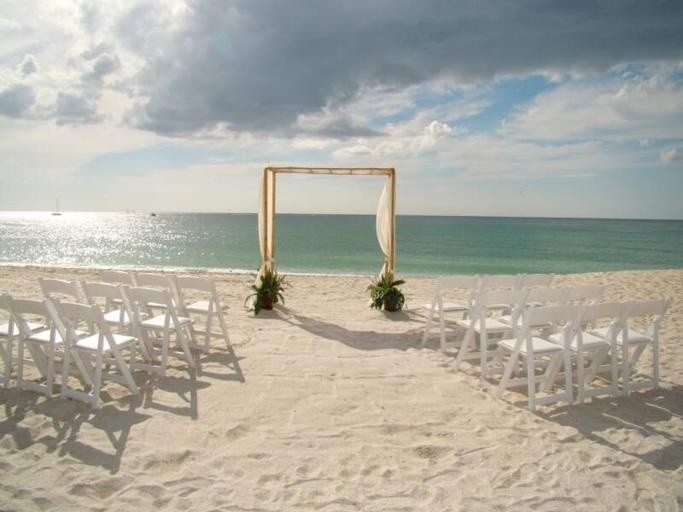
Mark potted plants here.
[364,271,406,312]
[243,264,292,314]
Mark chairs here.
[0,269,233,411]
[419,271,673,414]
